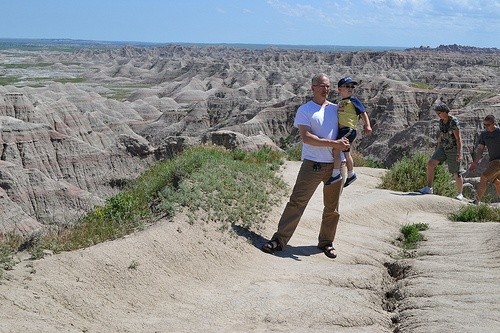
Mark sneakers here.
[326,174,342,184]
[343,173,357,187]
[419,186,433,194]
[455,192,463,201]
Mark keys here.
[313,163,320,172]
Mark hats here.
[338,76,357,88]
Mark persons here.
[467,113,500,206]
[262,73,351,258]
[418,104,464,201]
[324,77,372,188]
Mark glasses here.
[342,85,355,89]
[484,124,490,127]
[314,85,330,89]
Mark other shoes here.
[469,199,479,205]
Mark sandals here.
[262,238,283,254]
[318,243,337,258]
